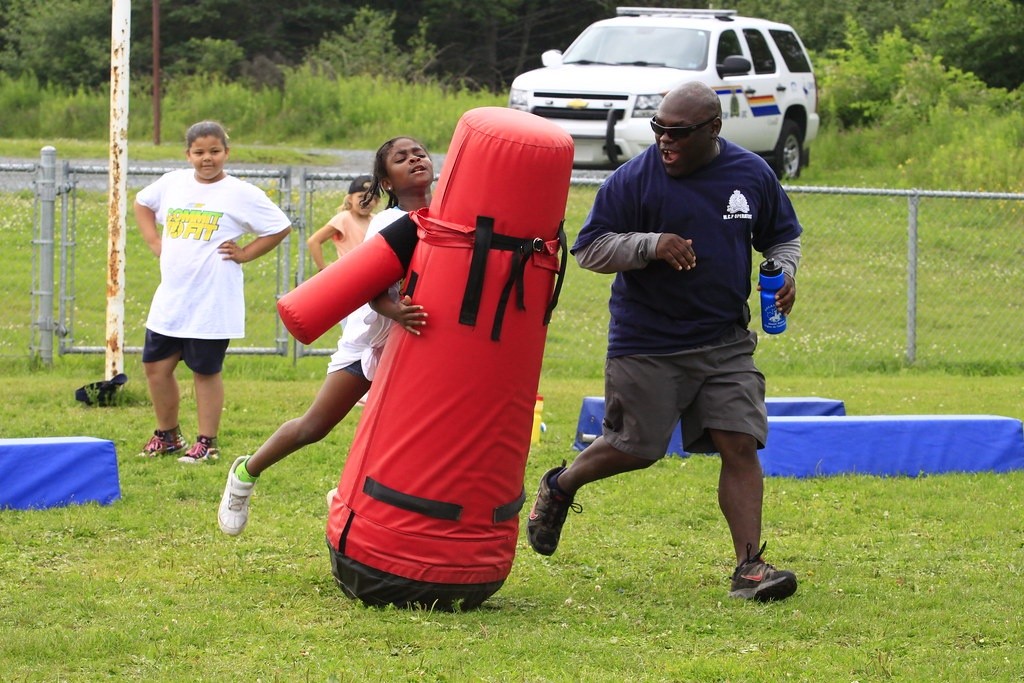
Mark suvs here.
[509,6,820,184]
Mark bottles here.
[759,258,787,334]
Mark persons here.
[218,137,434,536]
[133,121,293,462]
[528,81,804,604]
[306,175,382,406]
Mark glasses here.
[650,115,717,138]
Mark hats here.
[348,175,380,195]
[76,374,127,407]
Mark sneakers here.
[327,488,338,507]
[528,460,583,555]
[178,435,220,463]
[729,541,797,604]
[134,426,186,456]
[218,456,255,535]
